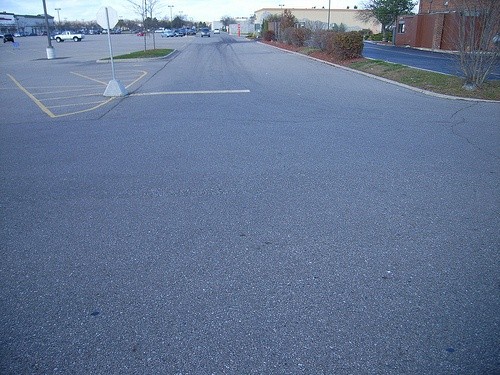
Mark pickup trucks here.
[54,31,85,43]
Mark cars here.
[136,32,144,36]
[155,28,196,38]
[80,30,121,34]
[201,29,210,38]
[3,34,14,43]
[214,29,220,34]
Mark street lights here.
[179,11,183,19]
[279,5,285,15]
[168,6,174,30]
[55,8,61,33]
